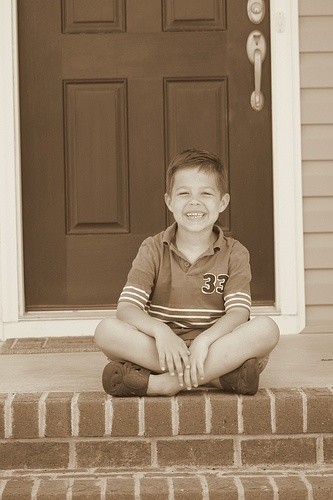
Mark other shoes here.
[216,357,259,395]
[101,359,151,398]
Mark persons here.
[92,146,280,399]
